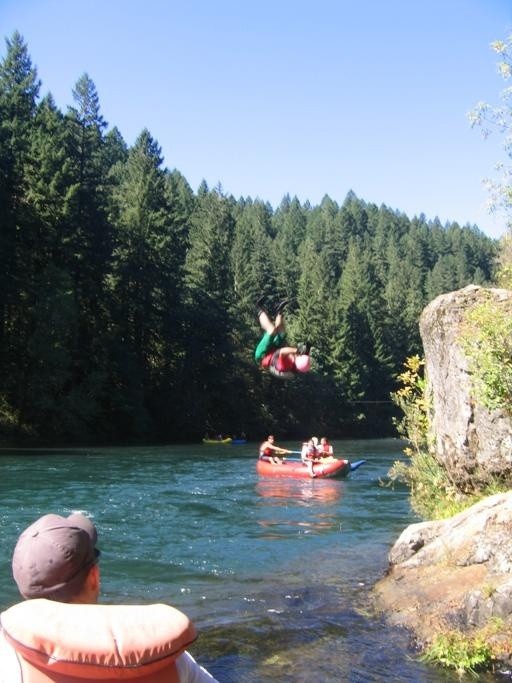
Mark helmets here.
[294,354,312,373]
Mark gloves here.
[296,340,311,354]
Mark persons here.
[319,436,333,458]
[258,435,288,465]
[301,439,321,477]
[0,513,218,682]
[252,296,313,379]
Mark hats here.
[10,510,103,605]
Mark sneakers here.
[252,293,290,317]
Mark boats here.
[202,438,247,446]
[255,459,351,480]
